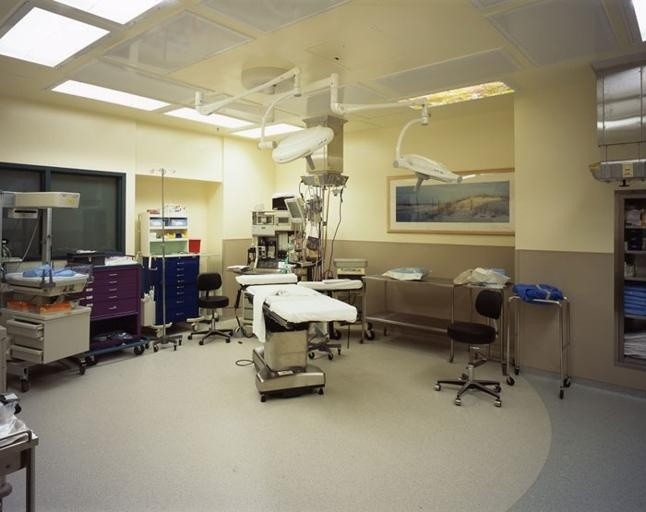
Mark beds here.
[236,271,359,401]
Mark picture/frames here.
[385,167,517,239]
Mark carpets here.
[0,314,559,512]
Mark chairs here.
[429,289,507,408]
[186,272,235,346]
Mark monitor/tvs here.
[284,198,304,224]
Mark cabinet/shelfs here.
[0,304,96,393]
[136,208,190,257]
[60,258,150,367]
[140,252,204,333]
[610,187,646,373]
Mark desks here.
[0,400,42,511]
[503,289,574,401]
[359,273,474,365]
[296,276,364,360]
[449,279,520,386]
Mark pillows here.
[235,274,301,289]
